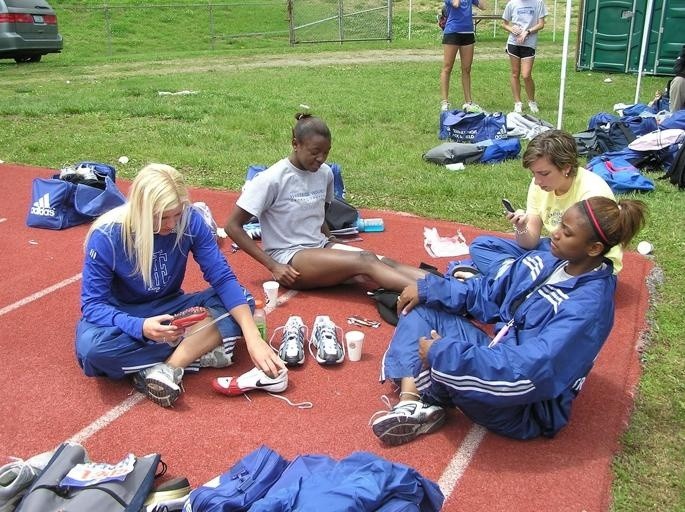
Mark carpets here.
[0,161,662,512]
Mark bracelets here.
[526,29,531,36]
[510,225,529,236]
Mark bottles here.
[356,218,385,232]
[250,300,268,340]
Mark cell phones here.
[502,199,520,220]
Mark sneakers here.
[0,452,56,512]
[164,306,209,348]
[513,102,524,113]
[200,345,236,368]
[310,315,345,366]
[441,102,451,111]
[450,264,481,282]
[277,316,306,367]
[129,362,185,409]
[211,363,289,398]
[462,103,482,112]
[372,400,448,446]
[138,477,193,512]
[528,101,539,113]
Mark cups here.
[345,330,364,363]
[636,242,654,256]
[264,281,279,308]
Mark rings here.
[396,296,401,303]
[162,337,167,341]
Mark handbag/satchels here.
[475,138,522,164]
[25,162,128,231]
[425,142,487,164]
[437,110,508,144]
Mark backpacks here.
[438,4,449,31]
[570,94,685,194]
[181,443,444,512]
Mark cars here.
[0,0,65,64]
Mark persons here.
[76,163,288,407]
[224,111,430,291]
[668,44,684,111]
[505,129,623,275]
[369,196,649,446]
[437,0,485,112]
[501,0,548,113]
